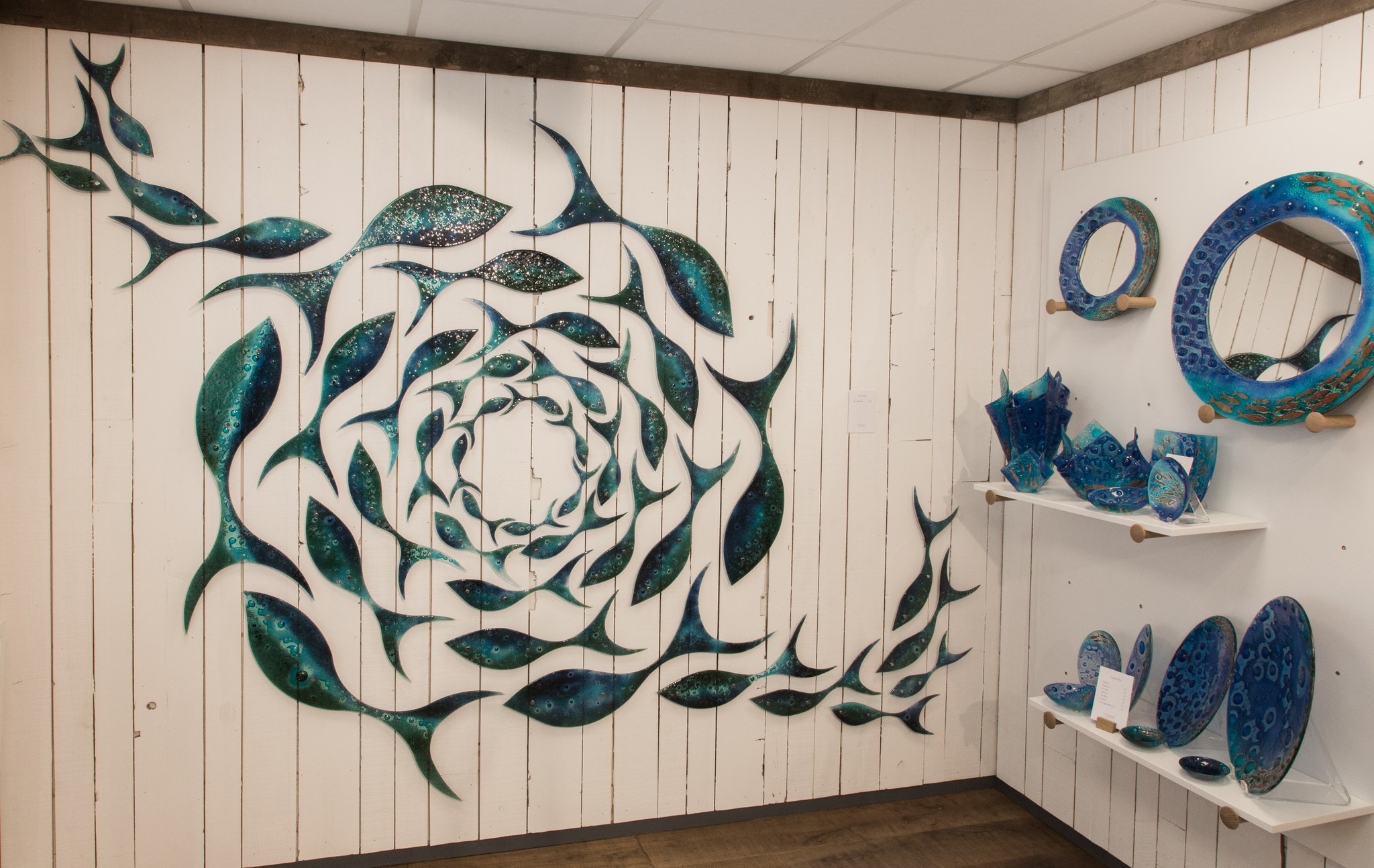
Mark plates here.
[1078,630,1121,690]
[1120,727,1164,749]
[1044,684,1096,711]
[1157,615,1237,748]
[1147,457,1189,521]
[1088,486,1147,513]
[1125,624,1153,712]
[1180,755,1231,780]
[1227,595,1315,794]
[1151,430,1218,514]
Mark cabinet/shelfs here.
[971,466,1374,868]
